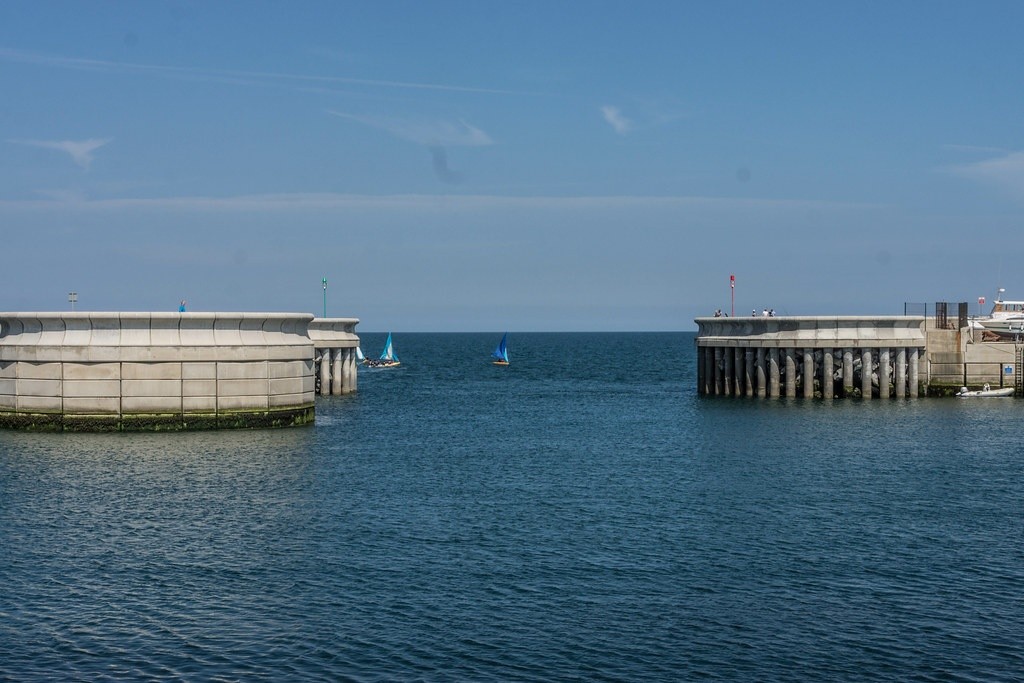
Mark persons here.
[713,308,722,317]
[751,310,756,317]
[762,309,775,317]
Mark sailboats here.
[369,332,401,367]
[491,331,510,366]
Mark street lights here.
[322,277,328,318]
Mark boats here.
[978,289,1024,333]
[955,384,1015,397]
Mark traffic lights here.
[730,276,735,317]
